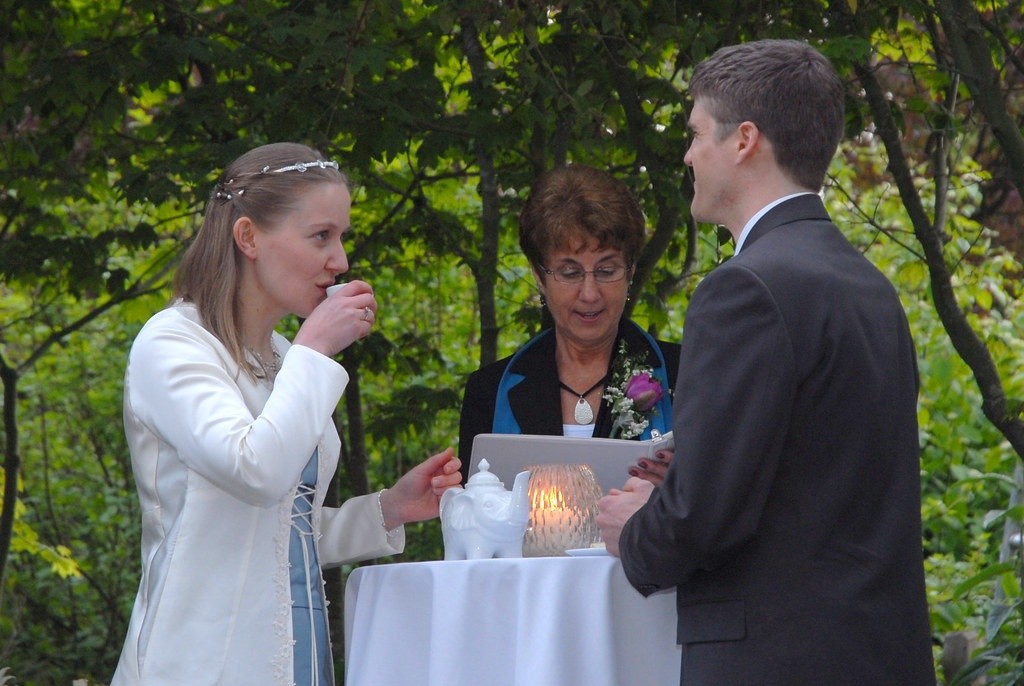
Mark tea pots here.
[438,458,531,560]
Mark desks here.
[343,557,683,686]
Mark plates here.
[565,547,614,557]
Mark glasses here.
[535,261,634,285]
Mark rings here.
[364,306,371,321]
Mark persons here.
[107,142,463,686]
[457,164,682,488]
[594,39,936,686]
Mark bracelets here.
[377,489,399,539]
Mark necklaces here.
[558,374,607,425]
[245,337,281,375]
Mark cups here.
[325,282,352,297]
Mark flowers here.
[602,338,675,441]
